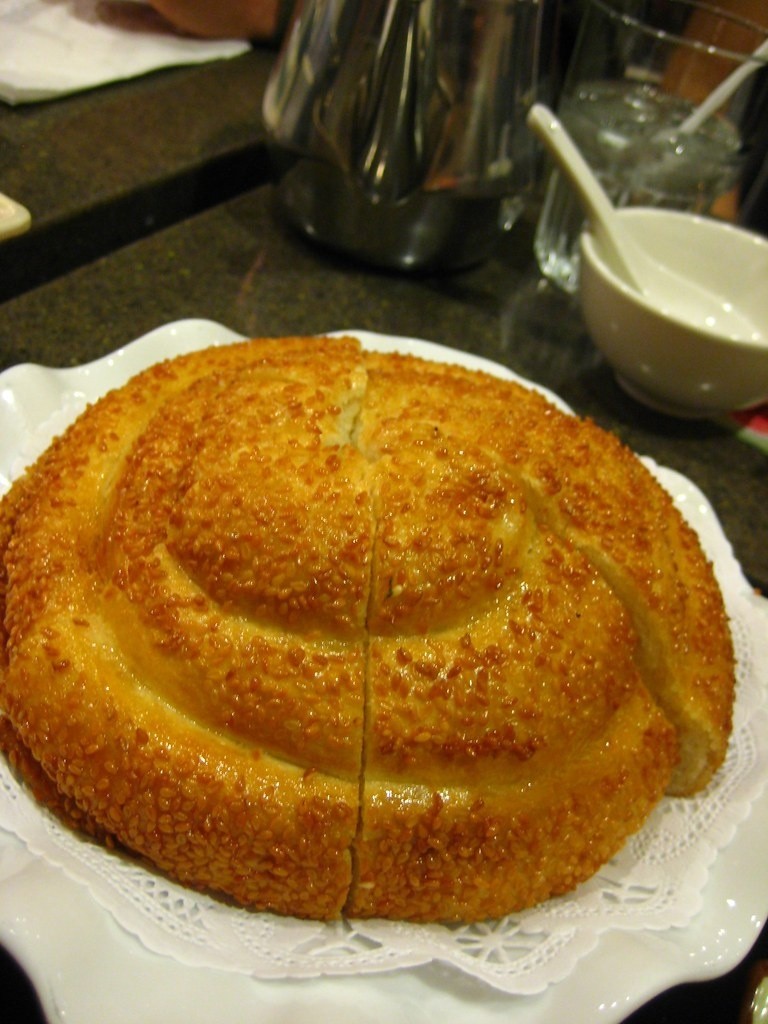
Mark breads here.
[0,331,736,926]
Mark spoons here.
[528,104,760,345]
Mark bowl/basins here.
[580,205,767,421]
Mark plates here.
[0,316,767,1024]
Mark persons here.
[44,0,280,41]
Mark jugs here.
[263,0,544,276]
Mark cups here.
[532,0,768,303]
